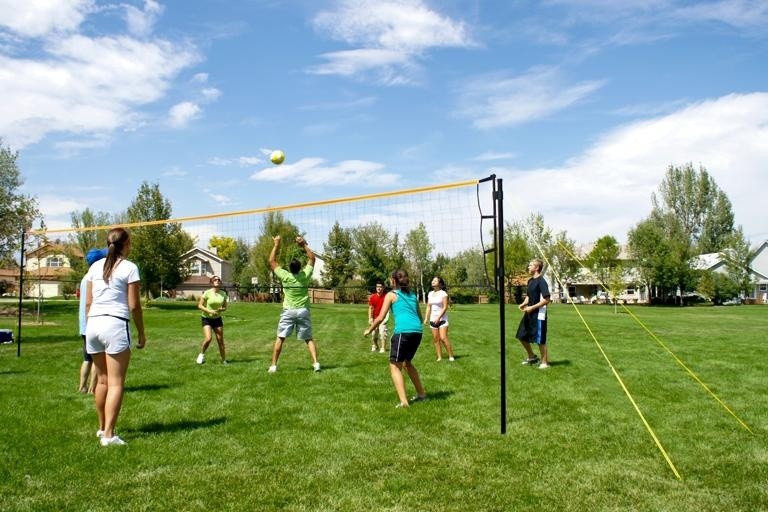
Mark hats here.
[85,247,109,267]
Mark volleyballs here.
[270,150,284,164]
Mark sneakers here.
[268,365,277,373]
[196,353,205,364]
[371,345,378,351]
[100,435,127,446]
[96,429,104,439]
[379,348,386,353]
[521,355,540,365]
[312,362,321,372]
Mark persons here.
[86,229,146,447]
[363,268,427,408]
[368,281,390,353]
[422,276,455,362]
[78,248,109,393]
[196,276,229,365]
[515,258,551,369]
[267,235,321,373]
